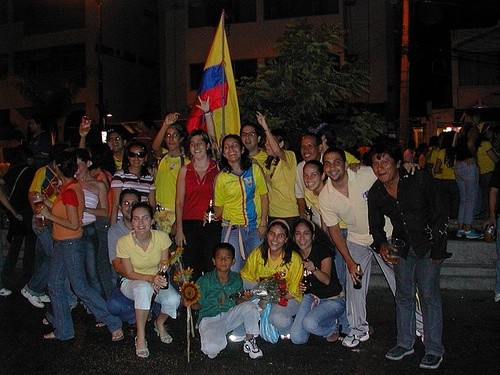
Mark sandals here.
[111,329,124,341]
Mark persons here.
[366,139,453,369]
[483,160,500,304]
[405,110,500,239]
[0,96,361,359]
[318,147,424,346]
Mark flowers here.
[229,272,290,306]
[161,247,202,363]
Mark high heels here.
[134,336,149,357]
[154,322,173,344]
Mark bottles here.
[304,206,313,221]
[302,268,310,295]
[205,199,215,227]
[159,264,170,291]
[353,264,362,289]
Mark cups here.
[81,115,94,134]
[383,237,405,264]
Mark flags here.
[185,15,241,145]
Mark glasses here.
[128,151,145,158]
[242,131,256,137]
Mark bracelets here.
[205,111,209,114]
[265,129,270,133]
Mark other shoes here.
[456,230,465,237]
[385,346,414,360]
[229,334,258,342]
[420,354,443,369]
[327,318,339,342]
[280,334,290,340]
[465,229,480,238]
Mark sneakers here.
[39,295,51,302]
[244,338,263,359]
[0,288,12,296]
[342,331,369,347]
[21,288,44,308]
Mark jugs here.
[33,193,48,229]
